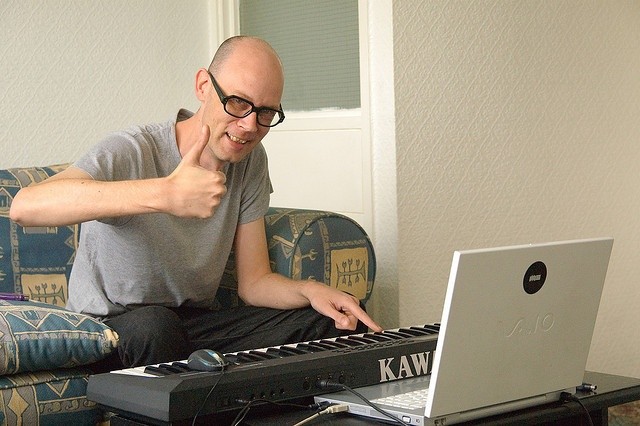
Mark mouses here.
[187,347,231,371]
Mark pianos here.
[86,323,443,421]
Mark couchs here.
[0,162,376,424]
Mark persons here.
[8,36,384,368]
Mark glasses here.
[208,69,285,127]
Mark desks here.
[97,370,639,424]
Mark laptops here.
[311,236,617,426]
